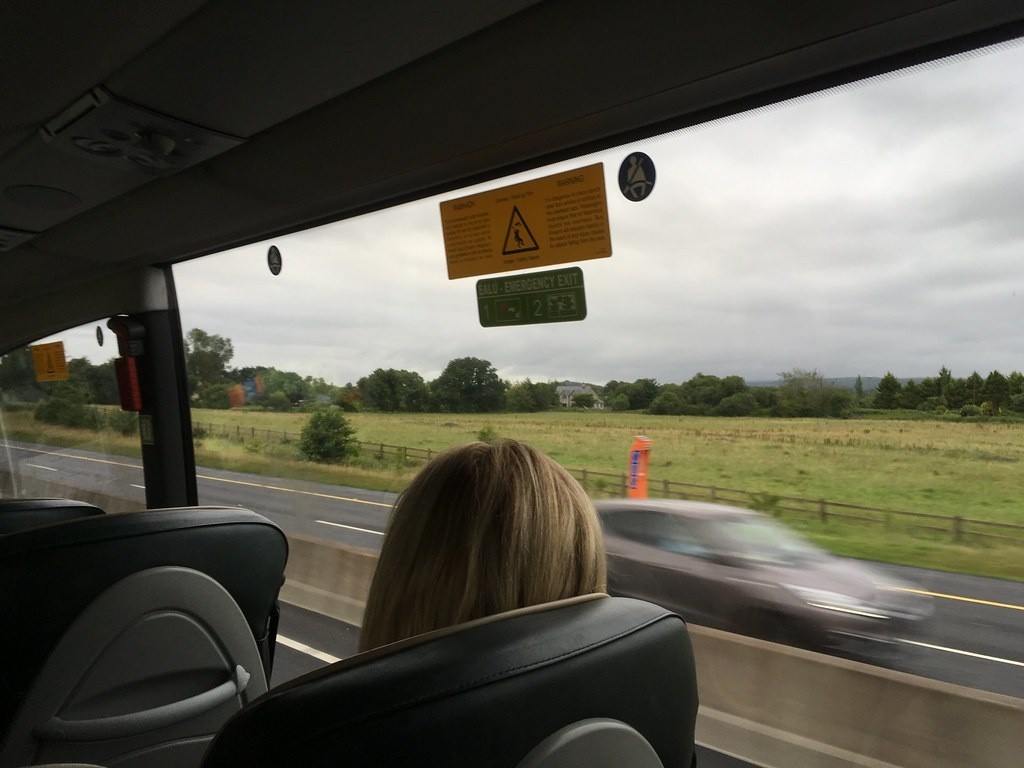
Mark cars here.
[588,496,937,662]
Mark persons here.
[358,438,608,652]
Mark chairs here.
[0,497,107,534]
[195,592,699,768]
[0,507,290,768]
